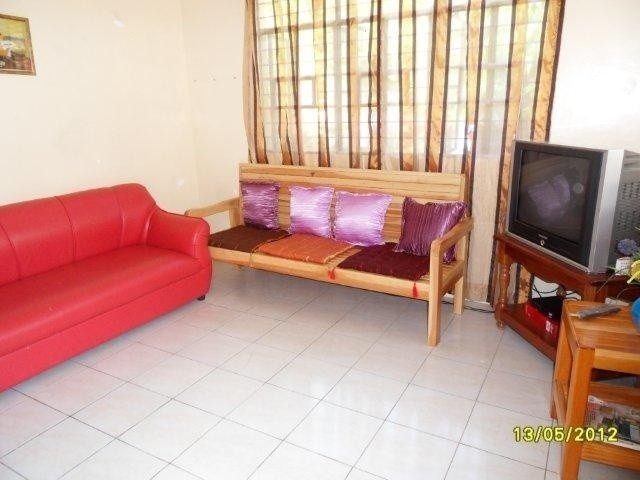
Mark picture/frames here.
[0,14,36,75]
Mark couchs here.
[185,163,474,346]
[0,183,213,393]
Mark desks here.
[550,299,640,480]
[493,233,640,381]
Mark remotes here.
[577,305,621,320]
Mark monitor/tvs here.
[504,139,640,274]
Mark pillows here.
[333,191,393,247]
[240,180,282,233]
[392,197,468,264]
[286,185,335,239]
